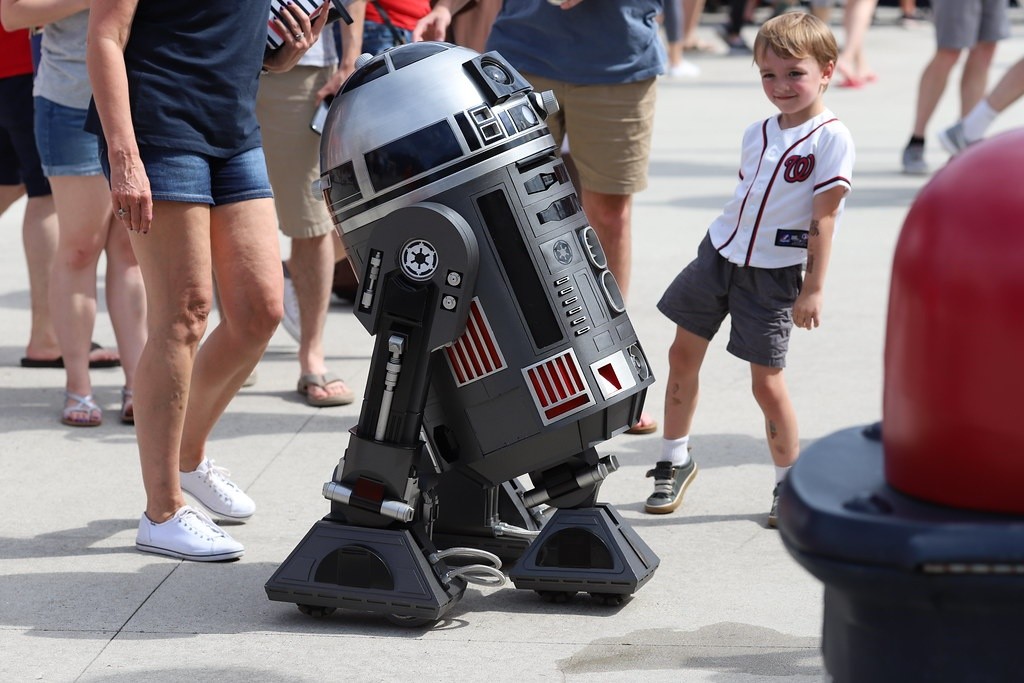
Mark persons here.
[88,0,334,562]
[937,57,1024,155]
[411,1,667,314]
[0,1,503,427]
[902,0,1009,175]
[643,12,854,528]
[657,0,916,89]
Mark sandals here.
[120,385,134,423]
[61,390,101,426]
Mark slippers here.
[20,342,120,368]
[297,372,353,406]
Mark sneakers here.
[768,482,780,527]
[135,505,244,561]
[179,456,256,520]
[645,447,699,514]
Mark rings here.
[117,209,130,217]
[295,32,305,41]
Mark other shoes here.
[715,25,752,56]
[280,260,303,344]
[670,60,697,75]
[903,15,931,31]
[938,119,985,158]
[835,73,875,87]
[902,144,927,174]
